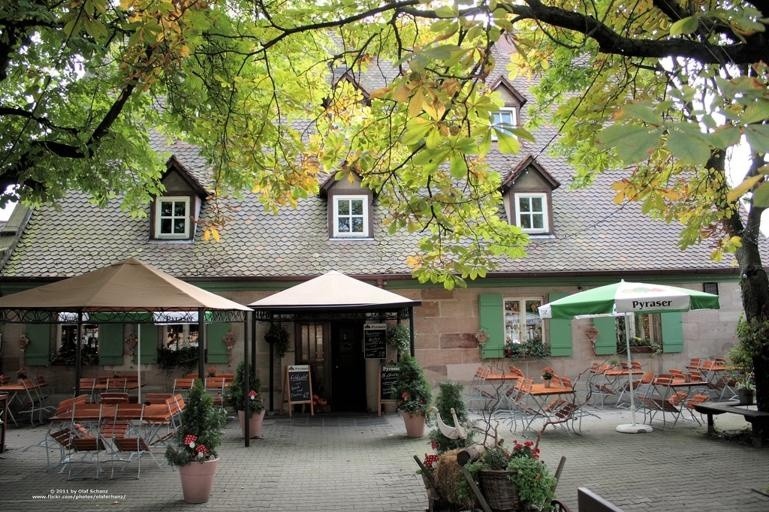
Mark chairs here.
[0,369,236,481]
[472,356,754,437]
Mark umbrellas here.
[538,278,721,427]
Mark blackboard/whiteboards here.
[289,372,310,401]
[364,330,387,359]
[379,364,401,403]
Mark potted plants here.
[232,362,265,441]
[394,349,556,511]
[165,374,228,504]
